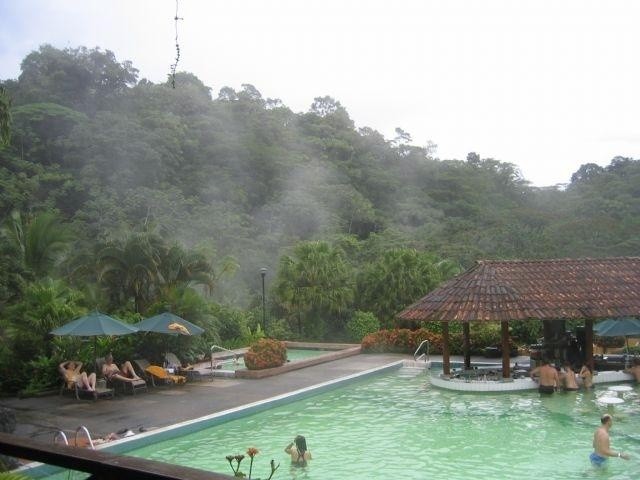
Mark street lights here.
[260,266,269,334]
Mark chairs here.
[60,359,213,403]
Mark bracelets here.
[617,452,620,457]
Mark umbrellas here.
[46,309,140,357]
[594,317,640,354]
[592,319,614,331]
[132,310,205,350]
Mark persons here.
[622,356,640,384]
[578,360,596,391]
[231,353,241,369]
[589,413,632,471]
[101,353,141,383]
[528,361,560,397]
[58,360,97,392]
[558,360,579,392]
[161,350,181,371]
[284,434,313,478]
